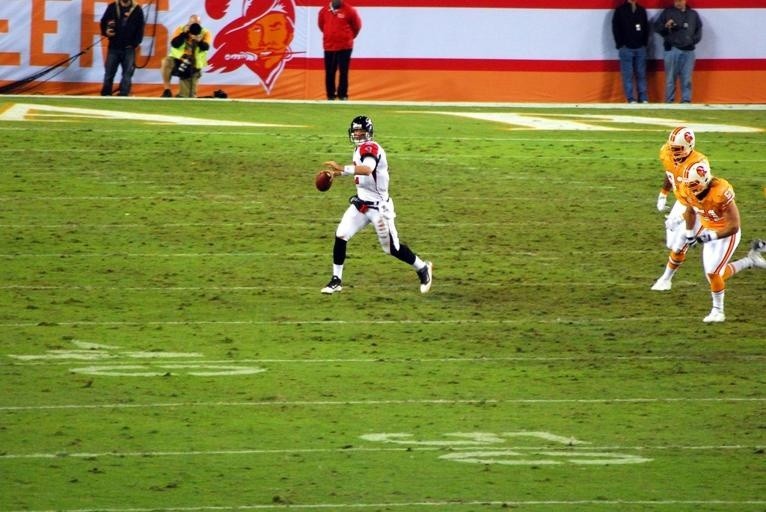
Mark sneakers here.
[650,277,672,291]
[416,260,432,293]
[163,89,172,97]
[748,248,766,267]
[320,276,342,293]
[702,308,725,322]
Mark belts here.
[362,200,378,205]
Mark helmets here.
[348,116,374,142]
[668,127,695,159]
[682,161,713,196]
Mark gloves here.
[657,197,670,211]
[695,234,712,244]
[664,215,682,231]
[686,236,698,246]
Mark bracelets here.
[343,165,355,175]
[340,171,348,176]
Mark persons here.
[653,1,703,104]
[318,0,362,101]
[320,116,434,296]
[649,125,711,291]
[99,1,145,97]
[213,0,295,93]
[159,15,213,99]
[678,160,766,322]
[612,1,650,104]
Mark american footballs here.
[315,172,333,192]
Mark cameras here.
[190,23,202,35]
